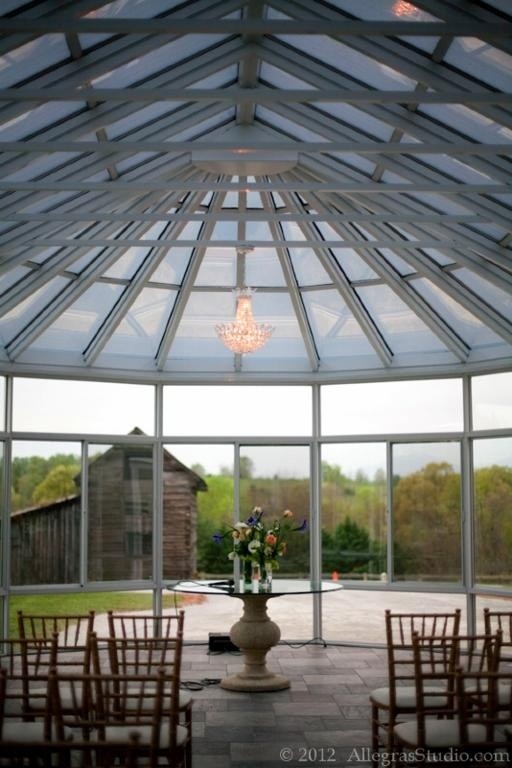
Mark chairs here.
[2,605,198,766]
[364,604,512,768]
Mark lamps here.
[213,243,276,355]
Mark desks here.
[164,576,343,696]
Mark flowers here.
[219,505,306,581]
